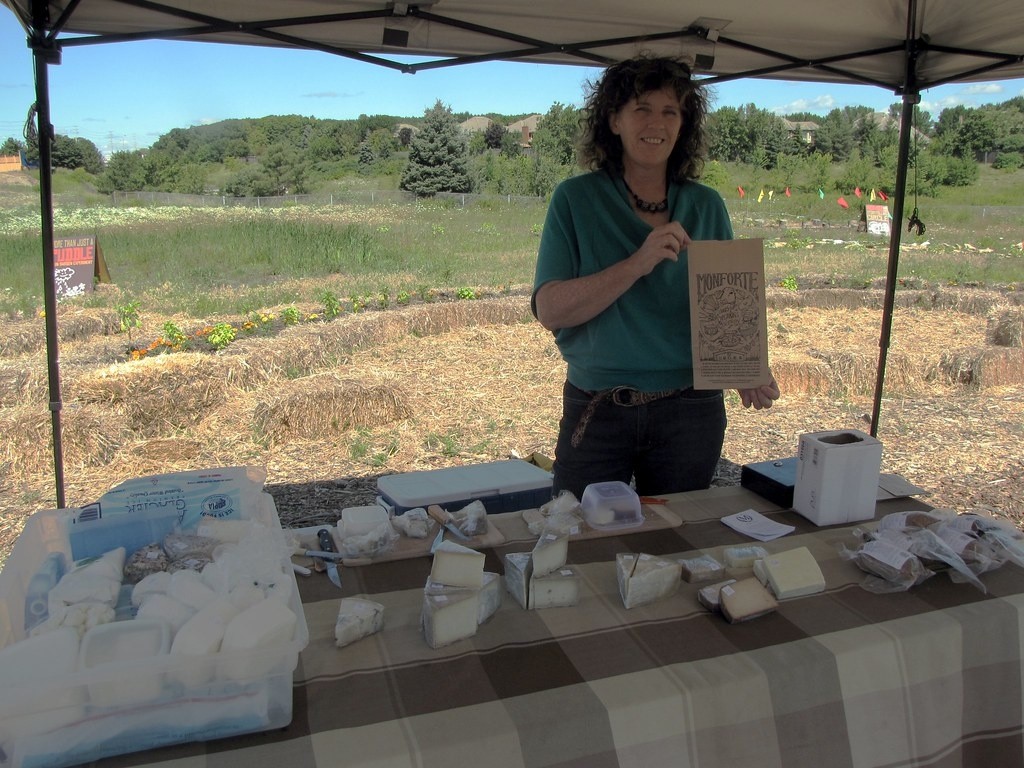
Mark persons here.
[532,58,780,498]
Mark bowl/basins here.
[582,481,645,531]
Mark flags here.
[870,189,876,202]
[758,190,764,203]
[877,191,889,201]
[738,186,744,198]
[768,191,773,200]
[855,187,862,198]
[785,187,791,197]
[818,189,824,199]
[837,197,848,208]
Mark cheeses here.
[528,494,584,537]
[419,529,584,650]
[617,546,825,623]
[336,598,385,648]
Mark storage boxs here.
[793,429,882,527]
[582,481,644,532]
[741,457,798,508]
[377,452,555,520]
[0,493,310,768]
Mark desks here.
[75,484,1024,767]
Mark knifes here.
[428,505,473,542]
[294,548,341,560]
[317,529,342,589]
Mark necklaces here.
[623,173,668,213]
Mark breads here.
[392,499,489,536]
[30,515,310,719]
